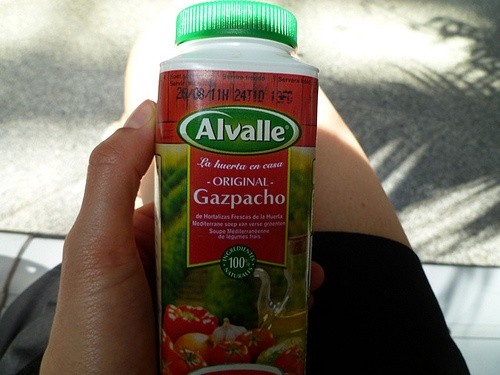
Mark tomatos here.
[161,303,304,375]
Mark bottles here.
[153,0,320,375]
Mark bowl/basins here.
[186,362,285,375]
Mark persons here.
[0,29,473,375]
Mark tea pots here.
[254,234,306,358]
